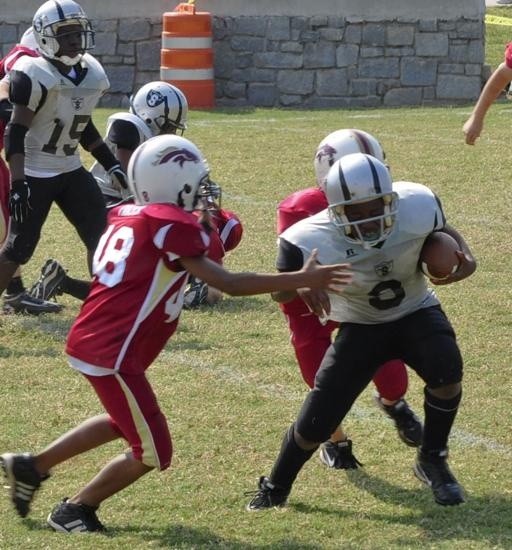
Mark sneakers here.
[3,289,62,316]
[183,276,208,310]
[413,445,465,505]
[46,497,107,532]
[244,476,290,511]
[1,452,50,518]
[319,436,362,469]
[372,384,423,447]
[30,259,68,303]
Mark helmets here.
[129,81,188,136]
[20,1,96,56]
[313,128,399,247]
[127,133,221,212]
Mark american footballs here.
[419,231,461,279]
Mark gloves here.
[106,164,129,202]
[8,180,33,224]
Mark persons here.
[90,81,188,208]
[0,0,126,299]
[30,206,243,311]
[247,152,476,515]
[0,26,61,311]
[0,135,353,533]
[463,44,512,146]
[278,129,428,469]
